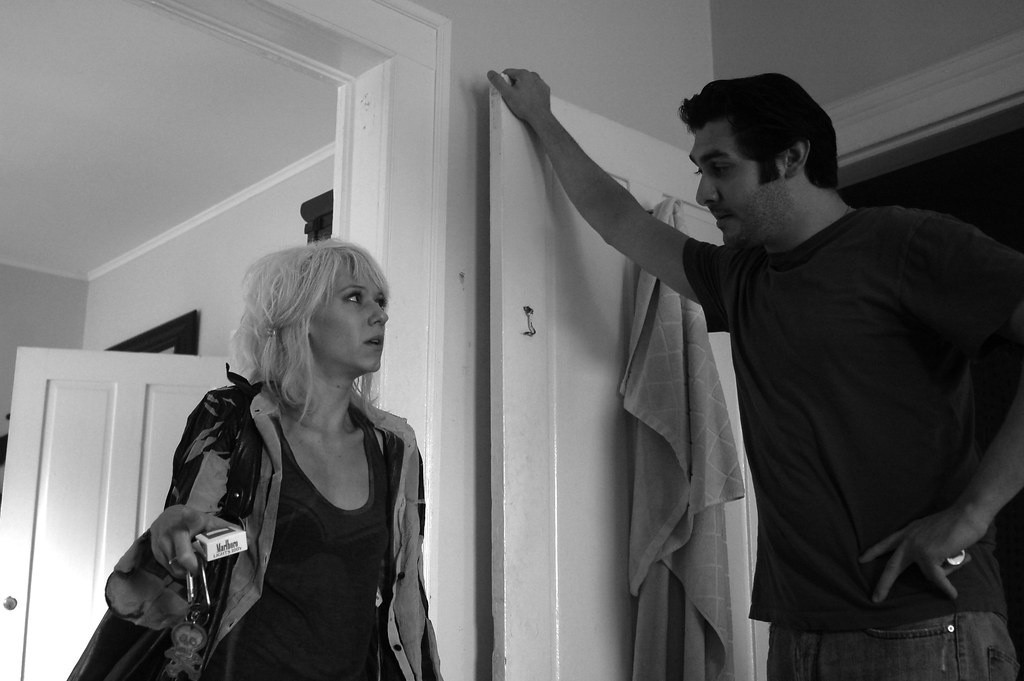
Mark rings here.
[167,556,177,565]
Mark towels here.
[618,198,746,680]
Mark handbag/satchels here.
[64,601,217,681]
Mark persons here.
[489,68,1023,681]
[107,242,443,681]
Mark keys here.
[165,552,214,680]
